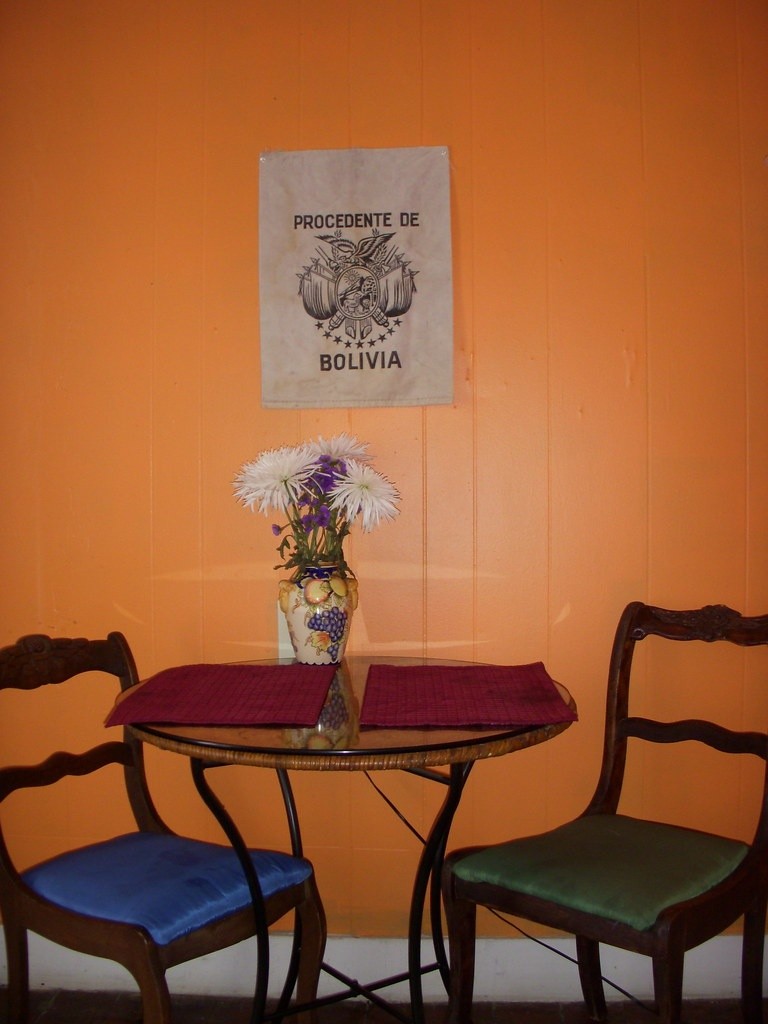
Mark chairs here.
[444,602,767,1023]
[1,632,327,1024]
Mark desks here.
[128,656,576,1024]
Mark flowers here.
[230,432,403,584]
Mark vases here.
[278,561,358,664]
[283,660,361,750]
